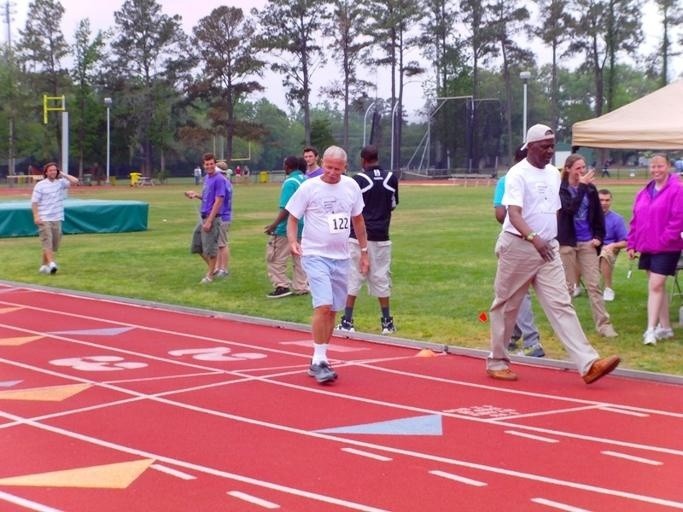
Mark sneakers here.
[380,317,397,334]
[217,270,228,278]
[573,285,582,298]
[213,268,219,275]
[200,277,214,285]
[264,285,292,299]
[642,330,656,346]
[525,345,544,358]
[38,265,49,274]
[306,361,339,384]
[602,325,618,338]
[655,322,676,340]
[602,287,614,301]
[334,318,356,333]
[48,262,57,275]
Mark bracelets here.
[360,245,368,253]
[526,230,537,241]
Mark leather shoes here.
[486,368,517,380]
[581,354,621,385]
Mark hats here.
[517,123,556,153]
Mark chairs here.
[667,251,683,305]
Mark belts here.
[504,230,532,244]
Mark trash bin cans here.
[259,171,268,184]
[83,173,92,186]
[111,175,116,186]
[130,172,142,188]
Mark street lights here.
[102,96,114,181]
[0,1,18,56]
[519,69,531,144]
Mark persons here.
[284,145,370,384]
[483,124,621,385]
[555,154,619,339]
[31,162,80,276]
[183,152,250,285]
[493,142,546,358]
[600,160,611,178]
[335,144,398,335]
[573,189,626,303]
[669,158,683,172]
[263,145,323,299]
[627,153,683,348]
